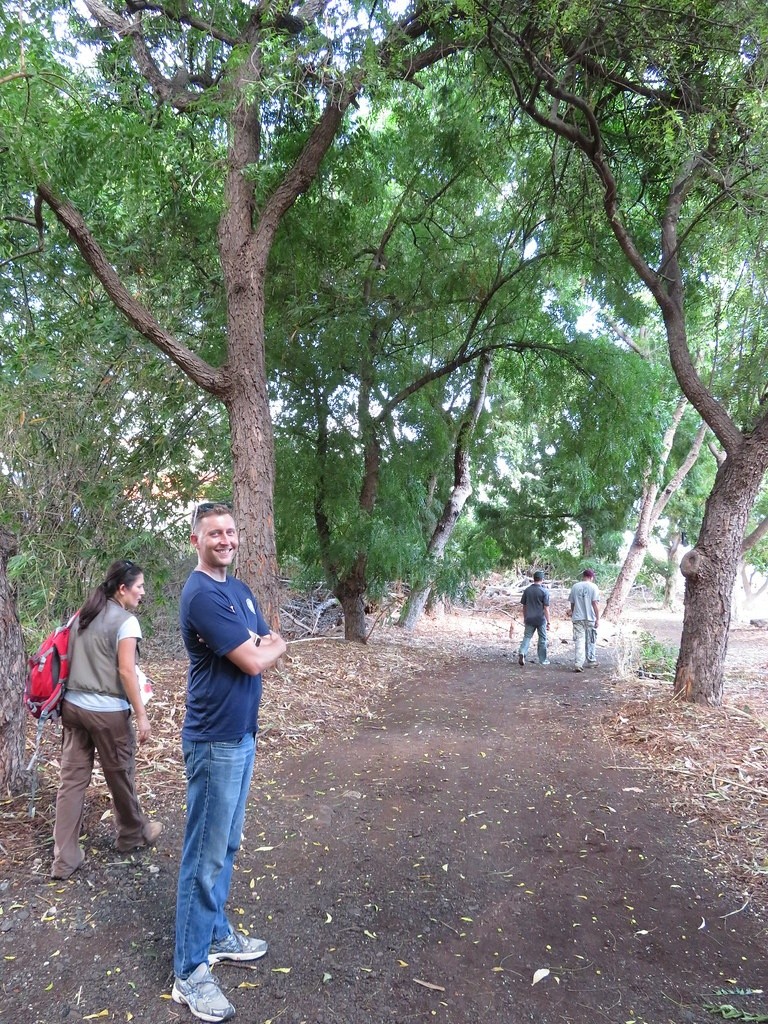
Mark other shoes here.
[142,822,163,844]
[79,849,85,863]
[576,667,585,673]
[589,663,599,669]
[540,659,551,665]
[519,654,524,666]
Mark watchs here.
[254,634,261,648]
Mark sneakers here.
[172,959,236,1022]
[207,924,270,965]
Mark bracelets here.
[547,623,550,624]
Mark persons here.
[567,569,600,672]
[518,571,550,666]
[50,560,164,880]
[170,503,287,1023]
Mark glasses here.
[193,503,234,533]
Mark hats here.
[584,570,596,578]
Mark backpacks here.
[24,607,83,722]
